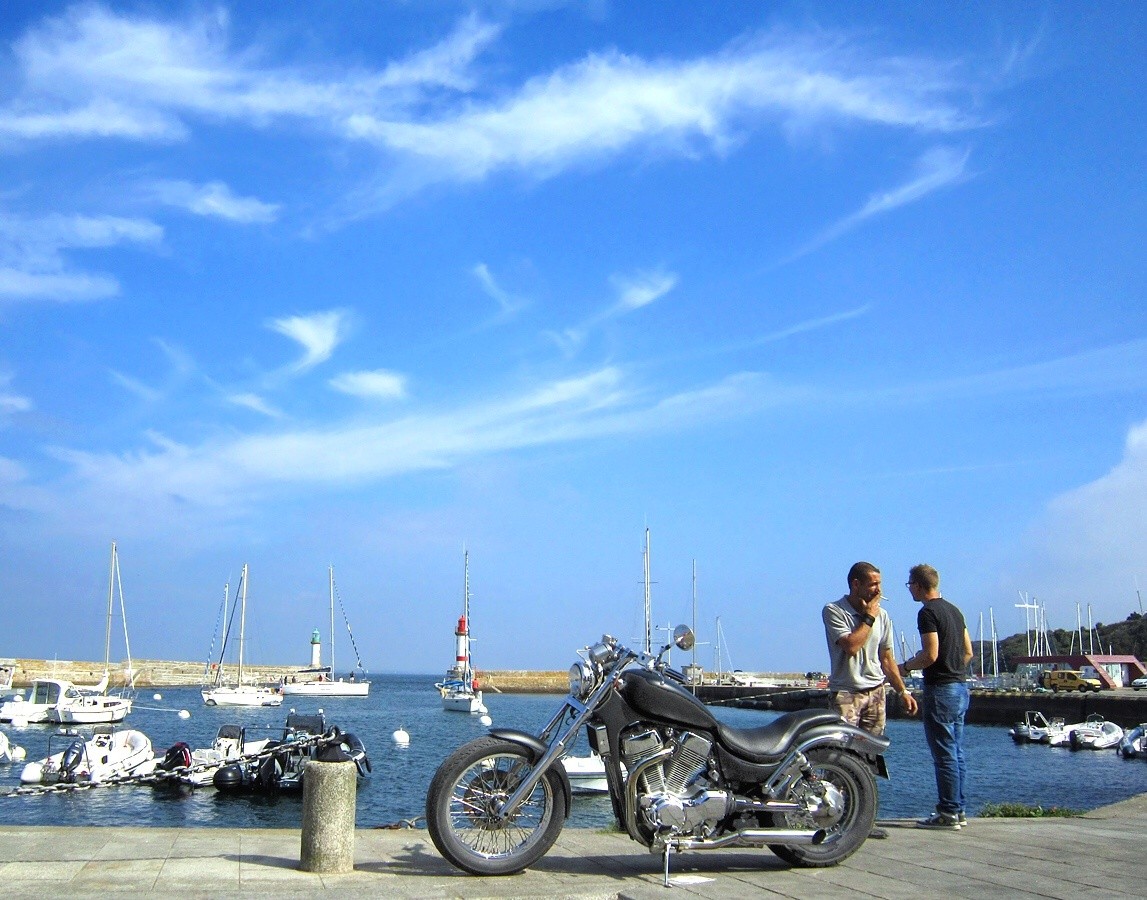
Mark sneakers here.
[916,811,962,831]
[929,811,968,825]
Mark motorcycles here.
[425,623,892,889]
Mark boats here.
[434,682,452,692]
[19,727,159,785]
[1008,718,1147,762]
[212,730,369,792]
[0,541,140,723]
[559,752,629,795]
[47,695,134,723]
[129,738,272,788]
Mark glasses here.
[905,582,915,588]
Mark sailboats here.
[277,567,369,697]
[442,549,483,713]
[201,564,284,708]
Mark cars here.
[1132,674,1147,691]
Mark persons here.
[897,564,974,830]
[822,562,918,839]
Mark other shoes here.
[869,828,889,839]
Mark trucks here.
[1043,669,1102,693]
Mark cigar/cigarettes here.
[880,596,889,601]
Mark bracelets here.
[897,689,912,703]
[903,662,911,672]
[862,614,875,627]
